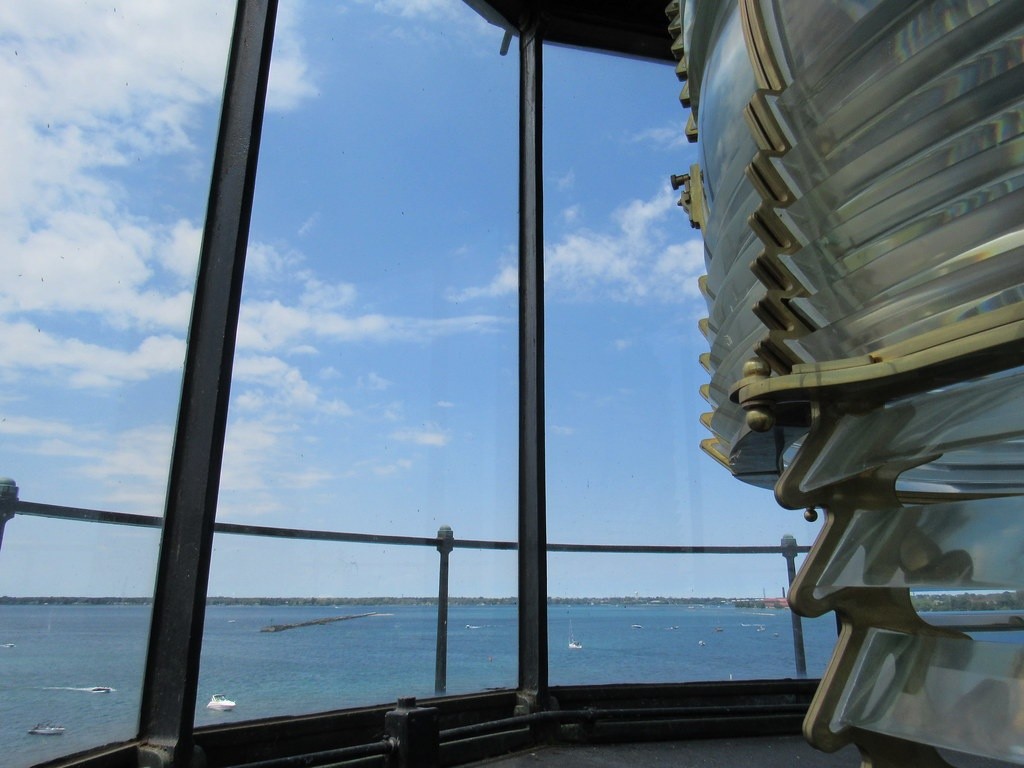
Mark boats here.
[698,640,706,645]
[568,641,583,649]
[716,626,723,632]
[466,625,470,628]
[90,688,111,694]
[206,694,236,712]
[741,612,781,636]
[27,721,66,735]
[670,624,679,629]
[2,643,14,648]
[631,624,642,628]
